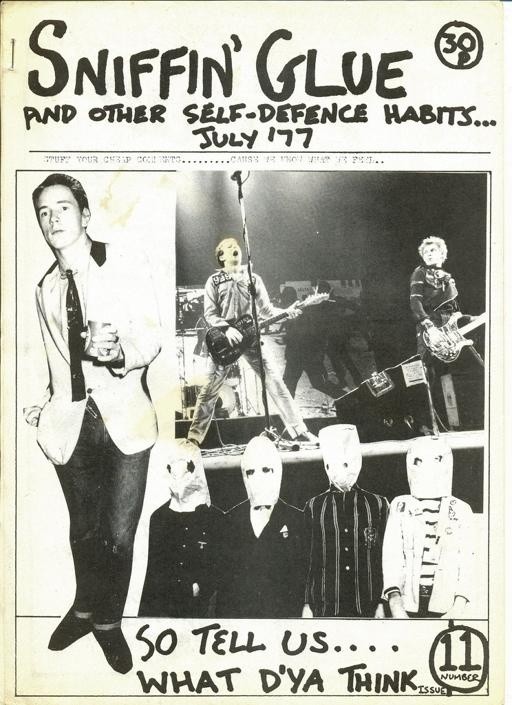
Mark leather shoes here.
[93,629,134,674]
[48,613,93,652]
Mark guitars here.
[206,291,330,367]
[418,310,486,366]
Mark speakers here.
[334,353,434,443]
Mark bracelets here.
[422,322,434,331]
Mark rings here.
[114,336,119,343]
[106,349,110,356]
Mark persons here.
[410,236,484,432]
[23,173,162,674]
[186,238,318,446]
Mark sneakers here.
[295,431,318,442]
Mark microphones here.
[230,171,242,181]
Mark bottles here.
[82,320,113,362]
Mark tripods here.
[235,181,301,451]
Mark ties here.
[65,269,86,404]
